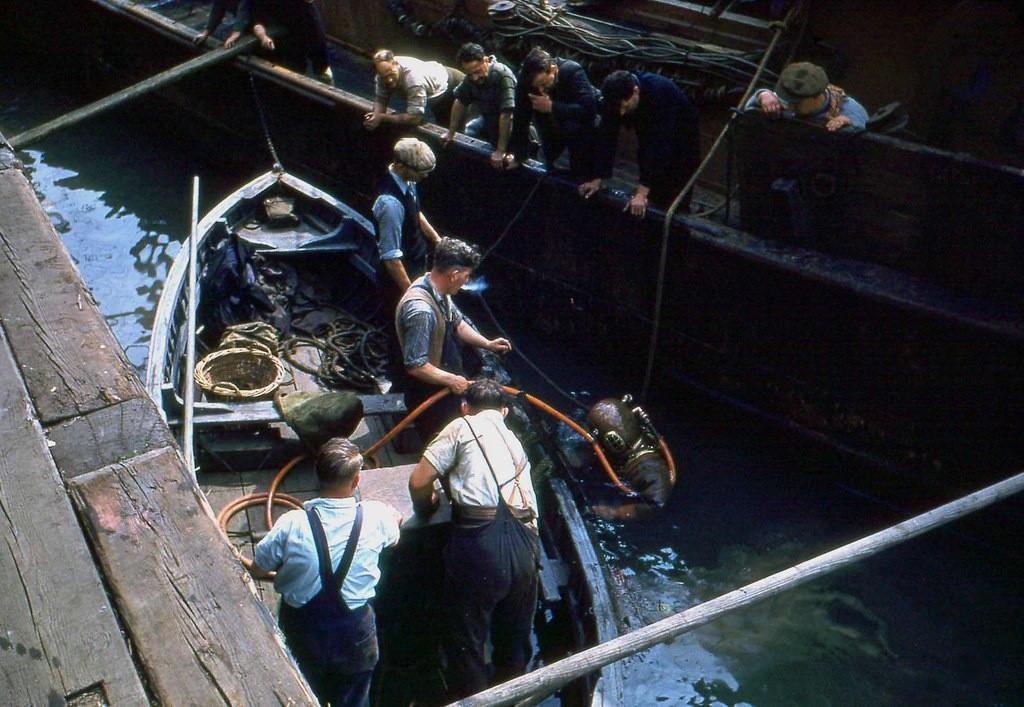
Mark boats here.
[141,159,624,706]
[89,0,1024,486]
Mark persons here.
[249,438,402,707]
[408,379,541,699]
[587,397,673,523]
[370,136,437,365]
[504,45,603,185]
[577,69,701,220]
[743,61,871,133]
[439,41,540,171]
[395,235,512,444]
[363,49,471,131]
[192,0,335,87]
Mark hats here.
[774,60,827,103]
[395,138,437,174]
